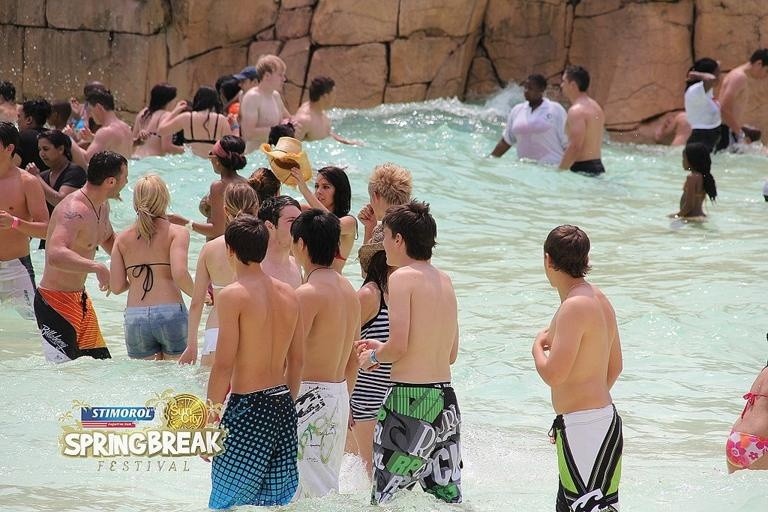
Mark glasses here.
[208,151,218,160]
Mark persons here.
[559,65,605,178]
[654,48,768,224]
[532,224,624,512]
[490,73,569,166]
[726,334,768,474]
[0,54,464,510]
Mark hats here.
[233,66,260,83]
[520,74,546,90]
[262,137,311,183]
[358,224,399,274]
